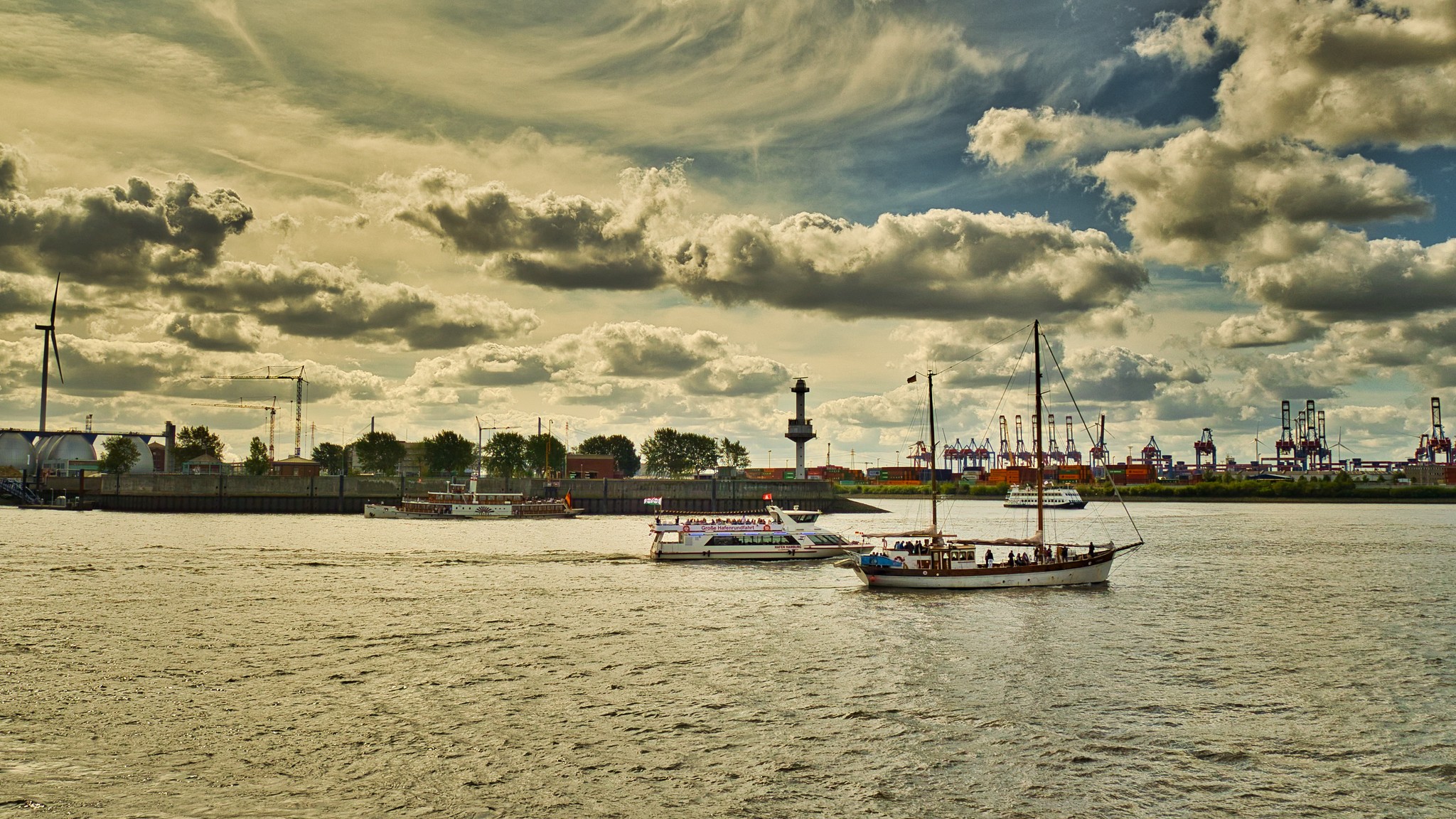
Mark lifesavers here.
[893,556,903,566]
[764,524,770,531]
[683,526,690,532]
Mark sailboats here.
[833,320,1149,588]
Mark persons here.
[985,545,1068,569]
[869,539,925,570]
[520,493,566,507]
[1088,542,1096,560]
[655,514,772,527]
[429,504,453,515]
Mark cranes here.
[200,365,310,458]
[191,396,281,476]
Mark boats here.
[1004,480,1089,509]
[364,473,584,520]
[644,493,876,560]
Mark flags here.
[644,497,655,505]
[860,532,864,535]
[650,497,662,506]
[565,488,572,510]
[855,532,859,534]
[763,494,772,500]
[907,374,917,384]
